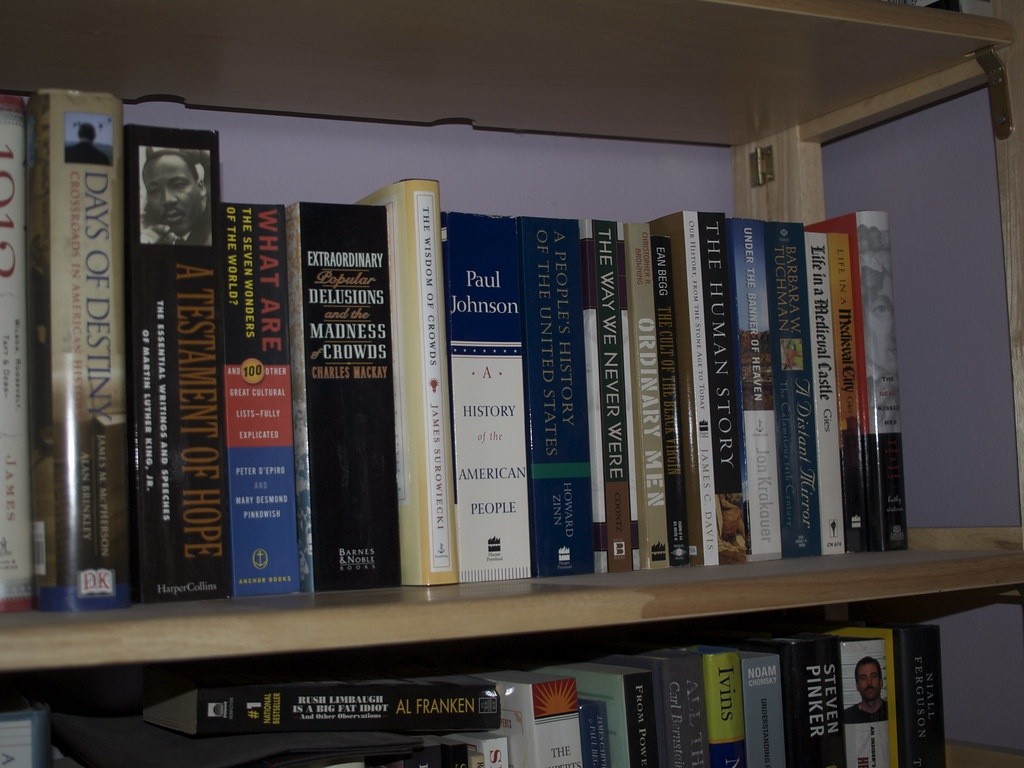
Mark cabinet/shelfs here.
[0,0,1024,768]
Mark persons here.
[142,151,213,246]
[846,657,888,723]
[65,122,112,165]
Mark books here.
[0,625,943,768]
[0,89,907,608]
